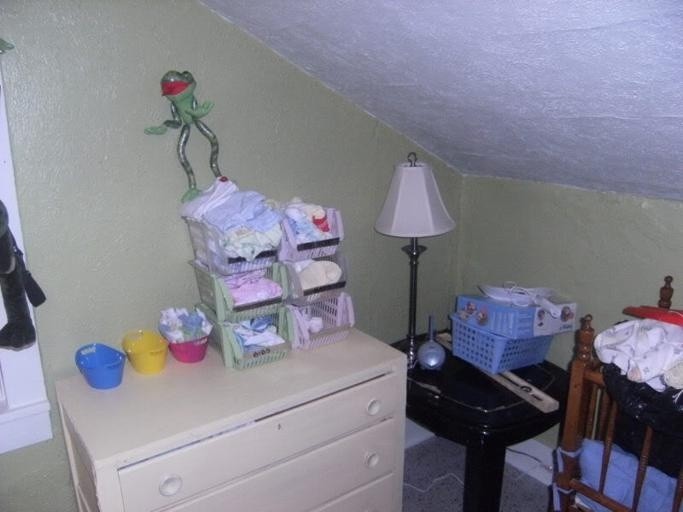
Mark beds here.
[548,273,683,512]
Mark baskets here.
[449,313,553,374]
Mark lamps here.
[373,152,457,370]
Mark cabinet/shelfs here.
[53,327,408,512]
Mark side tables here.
[389,330,572,511]
